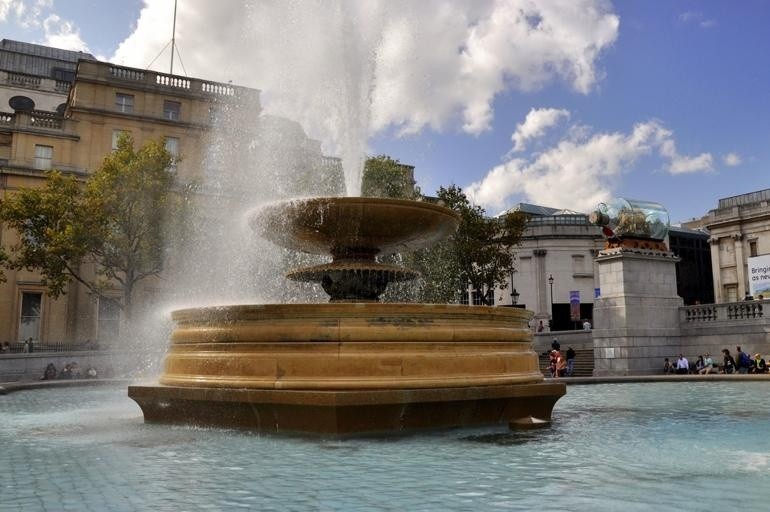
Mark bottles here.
[589,196,671,240]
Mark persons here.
[742,290,756,318]
[43,361,98,379]
[757,295,764,316]
[29,336,33,352]
[2,341,10,352]
[23,340,29,353]
[546,337,576,378]
[582,318,591,330]
[537,320,544,332]
[663,345,770,374]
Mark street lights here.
[510,288,519,306]
[548,274,553,303]
